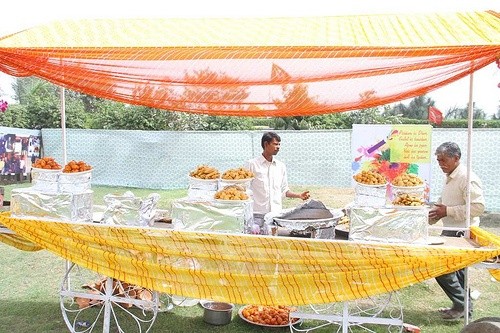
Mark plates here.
[238,305,302,327]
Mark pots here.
[202,302,235,325]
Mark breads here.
[392,172,424,187]
[215,188,249,200]
[392,193,425,206]
[354,170,386,185]
[63,160,91,173]
[221,167,253,179]
[190,164,219,179]
[242,305,299,325]
[32,157,62,169]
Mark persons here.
[429,141,484,319]
[0,134,41,174]
[243,132,311,236]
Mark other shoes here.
[438,307,453,313]
[442,307,472,321]
